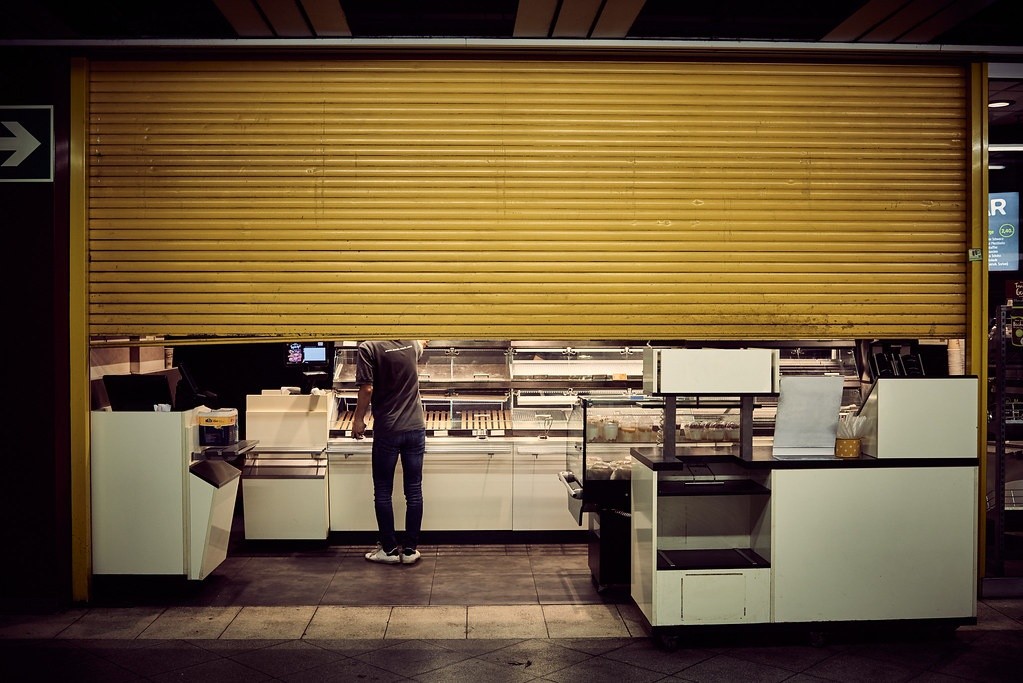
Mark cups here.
[603,422,619,443]
[620,416,636,441]
[587,423,599,442]
[639,417,654,442]
[836,439,862,458]
[684,420,740,441]
[165,348,174,369]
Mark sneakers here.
[365,541,401,565]
[402,548,421,565]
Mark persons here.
[352,340,426,565]
